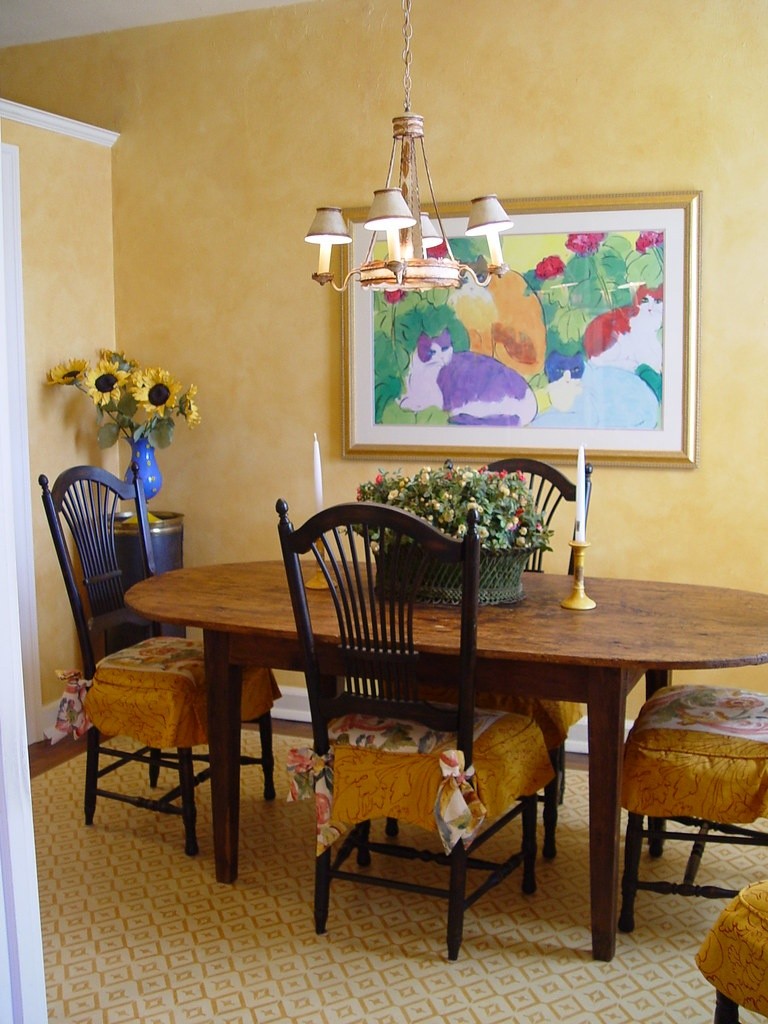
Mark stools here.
[616,683,768,925]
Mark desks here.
[124,562,768,957]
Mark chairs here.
[382,459,593,859]
[38,461,276,856]
[276,498,537,960]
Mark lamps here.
[302,0,512,296]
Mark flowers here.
[350,462,552,554]
[46,349,202,449]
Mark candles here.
[576,444,587,546]
[312,432,324,514]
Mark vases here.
[119,437,163,524]
[374,545,546,609]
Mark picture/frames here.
[335,191,701,468]
